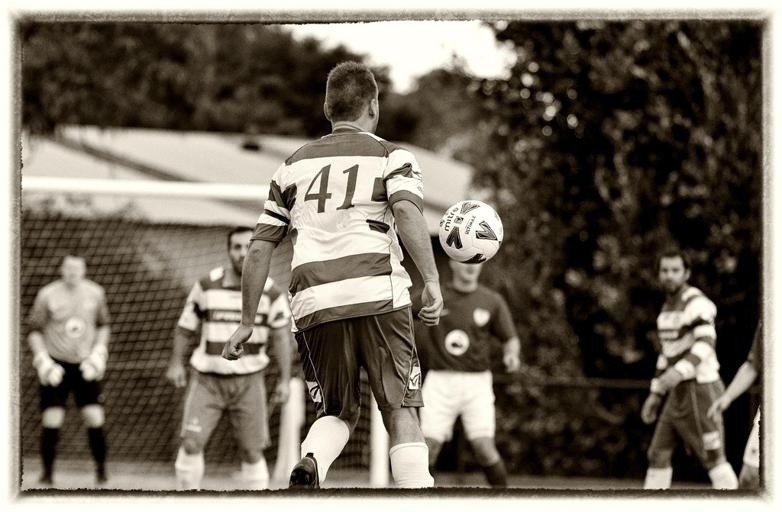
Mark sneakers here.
[289,451,322,491]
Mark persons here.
[165,226,294,490]
[26,253,114,484]
[220,60,445,488]
[640,249,739,490]
[706,317,762,487]
[410,258,522,488]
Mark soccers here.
[438,199,504,264]
